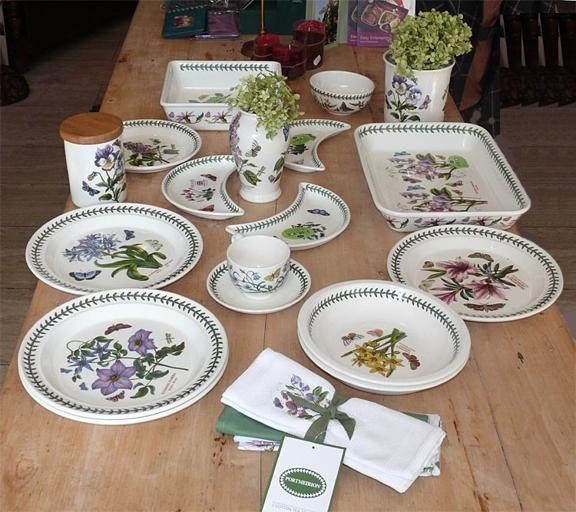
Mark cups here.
[59,112,127,203]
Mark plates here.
[285,119,351,173]
[387,223,564,322]
[297,280,471,396]
[123,118,202,174]
[25,203,204,295]
[18,288,229,426]
[353,122,532,233]
[206,258,312,314]
[162,154,244,220]
[225,182,351,250]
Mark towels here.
[217,347,446,494]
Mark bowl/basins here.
[226,235,290,297]
[160,59,282,132]
[308,69,375,116]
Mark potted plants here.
[383,9,473,123]
[228,69,305,203]
